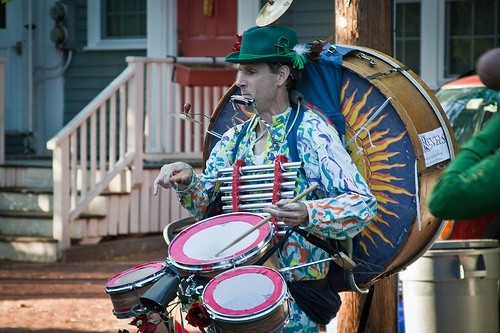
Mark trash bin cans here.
[402,239,500,333]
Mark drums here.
[104,260,167,320]
[201,265,292,333]
[165,211,281,294]
[202,44,459,294]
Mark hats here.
[224,24,311,69]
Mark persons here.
[153,24,378,333]
[427,109,500,220]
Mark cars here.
[433,74,500,239]
